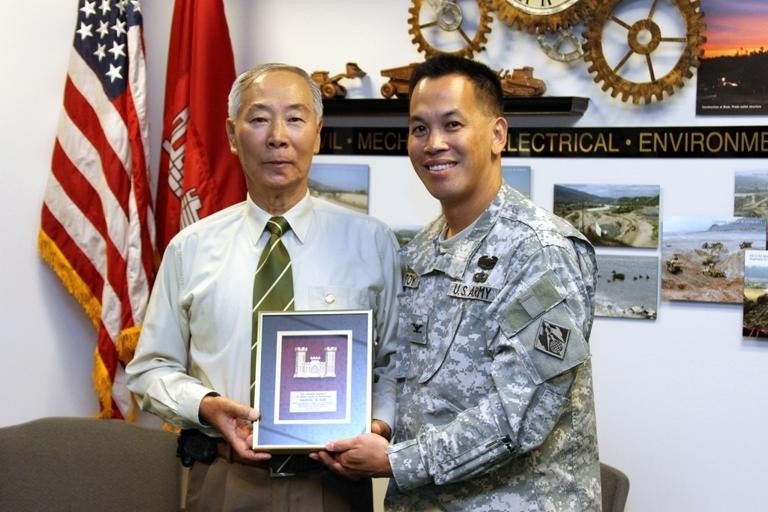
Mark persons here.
[125,63,400,507]
[308,53,604,508]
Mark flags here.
[153,0,247,257]
[37,0,183,435]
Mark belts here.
[215,442,324,478]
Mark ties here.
[250,215,294,408]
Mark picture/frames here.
[253,309,370,449]
